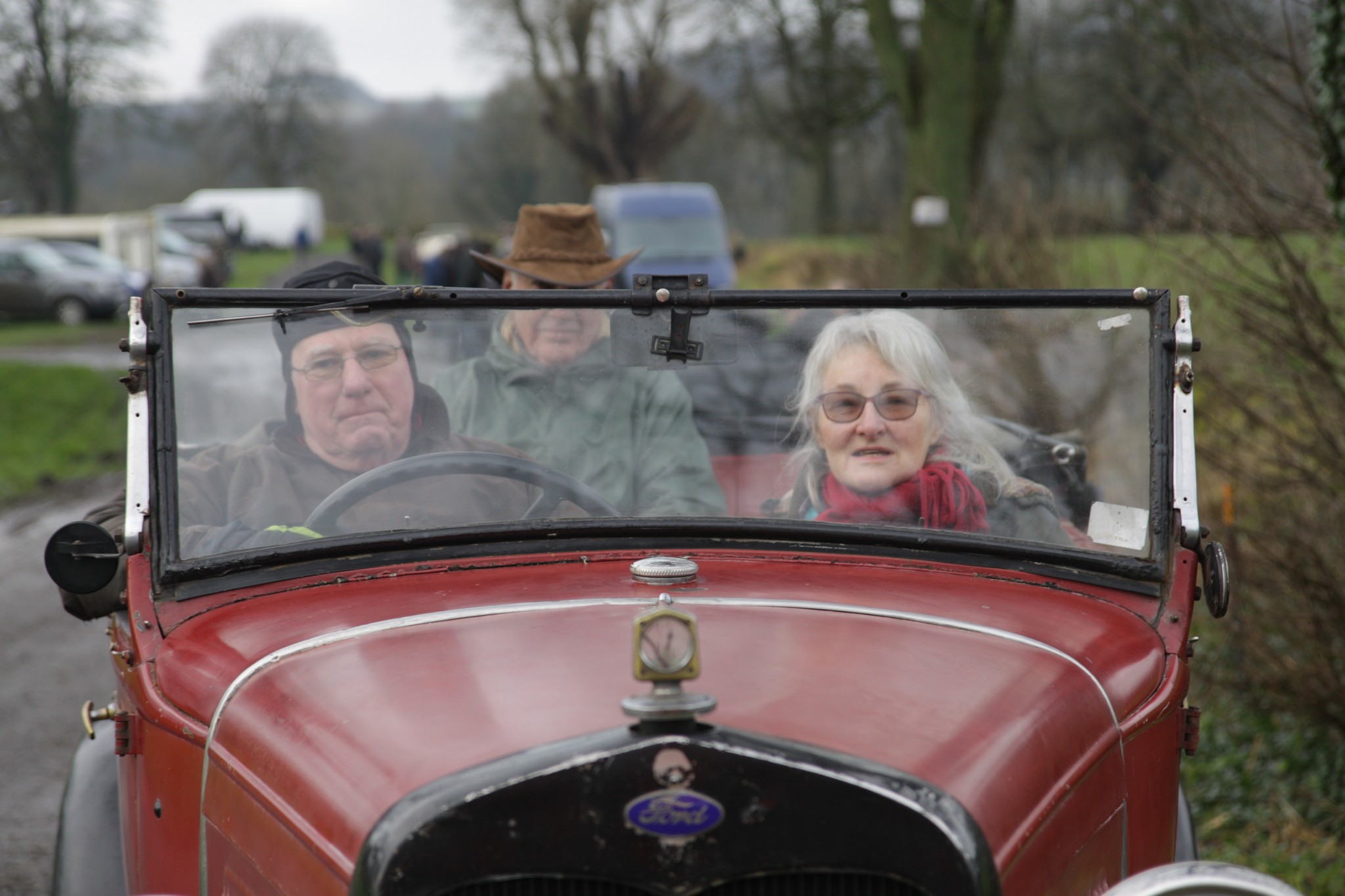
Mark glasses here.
[287,340,407,378]
[817,387,933,424]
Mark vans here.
[590,181,747,292]
[179,188,328,254]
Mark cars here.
[1,204,231,333]
[42,267,1307,896]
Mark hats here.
[471,202,644,287]
[274,261,411,362]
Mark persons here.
[179,261,587,560]
[430,203,729,519]
[773,308,1073,546]
